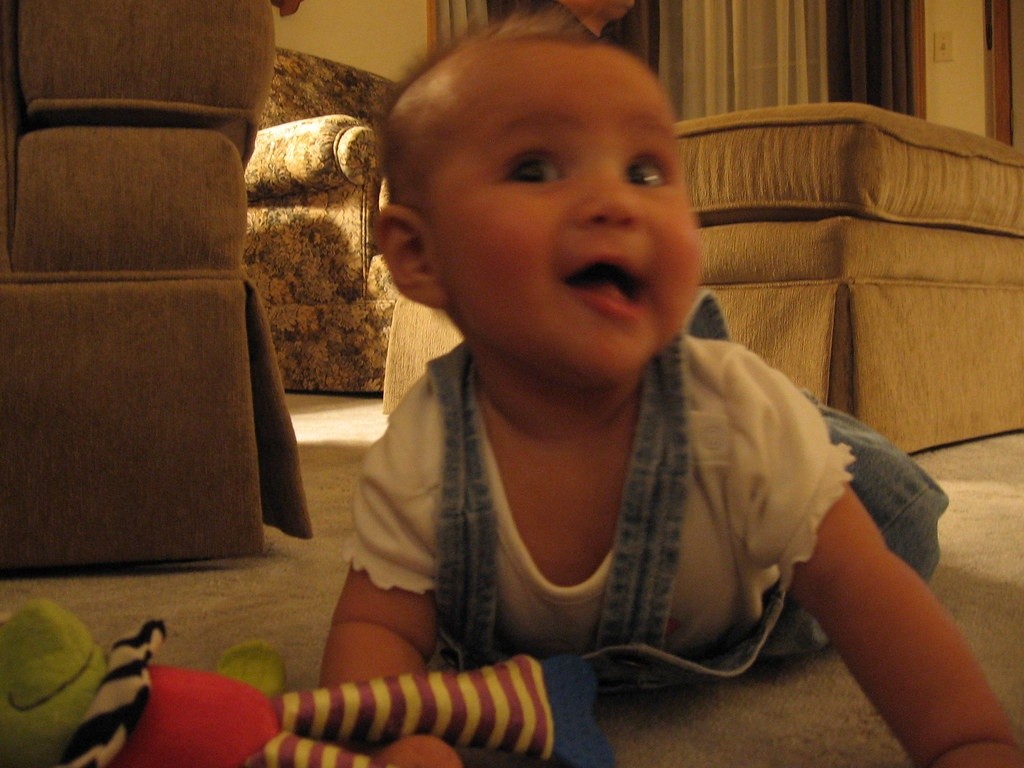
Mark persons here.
[317,1,1024,768]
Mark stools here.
[671,102,1024,454]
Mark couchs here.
[0,0,396,580]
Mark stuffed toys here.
[0,594,621,767]
